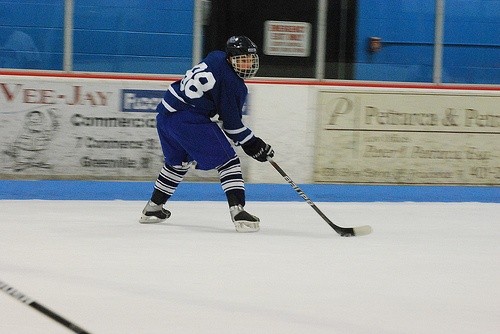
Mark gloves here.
[242,136,275,163]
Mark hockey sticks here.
[263,152,374,237]
[0,280,91,334]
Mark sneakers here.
[140,200,171,223]
[229,203,260,232]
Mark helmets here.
[225,36,260,79]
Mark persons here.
[139,37,274,232]
[1,16,83,72]
